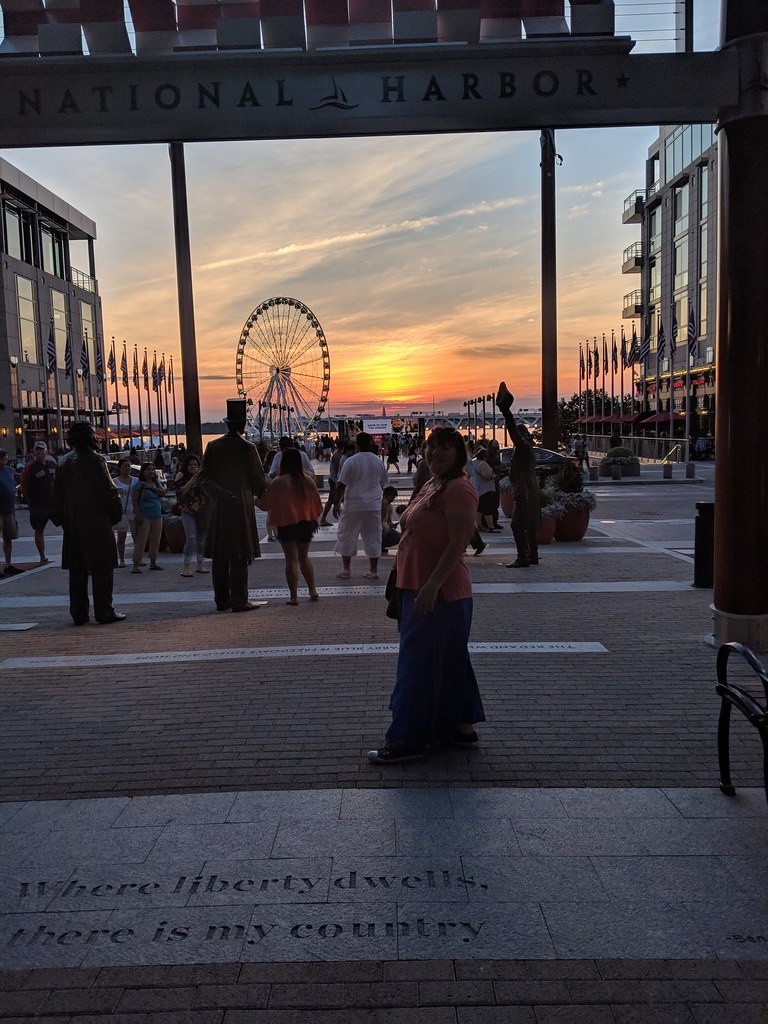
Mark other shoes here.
[486,523,504,533]
[216,601,260,612]
[337,569,351,577]
[0,565,24,579]
[267,536,278,542]
[505,551,539,567]
[309,591,320,601]
[119,560,211,576]
[73,611,126,626]
[363,572,378,579]
[286,598,299,606]
[40,558,48,565]
[473,543,489,556]
[319,521,333,526]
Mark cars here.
[499,445,583,489]
[14,459,167,506]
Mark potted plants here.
[160,499,187,553]
[599,447,640,476]
[499,460,599,545]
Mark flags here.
[579,308,696,380]
[47,323,171,394]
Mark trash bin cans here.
[691,499,712,593]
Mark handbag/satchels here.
[111,514,129,532]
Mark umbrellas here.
[96,428,162,439]
[574,410,684,423]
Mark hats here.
[33,441,47,449]
[223,398,252,421]
[0,448,8,455]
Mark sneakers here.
[438,731,480,744]
[366,747,424,764]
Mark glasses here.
[143,461,154,464]
[431,423,456,435]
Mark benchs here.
[715,641,768,805]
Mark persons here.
[314,433,432,580]
[496,383,541,569]
[196,399,267,613]
[696,433,706,451]
[255,436,324,605]
[463,432,504,556]
[20,441,59,566]
[610,430,623,449]
[367,427,486,765]
[111,440,120,452]
[124,440,130,450]
[114,443,209,578]
[0,448,25,577]
[565,434,590,472]
[49,421,125,625]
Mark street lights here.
[278,403,286,438]
[478,395,486,440]
[269,402,277,443]
[486,393,496,439]
[463,400,470,442]
[288,405,294,440]
[259,400,268,440]
[470,398,478,442]
